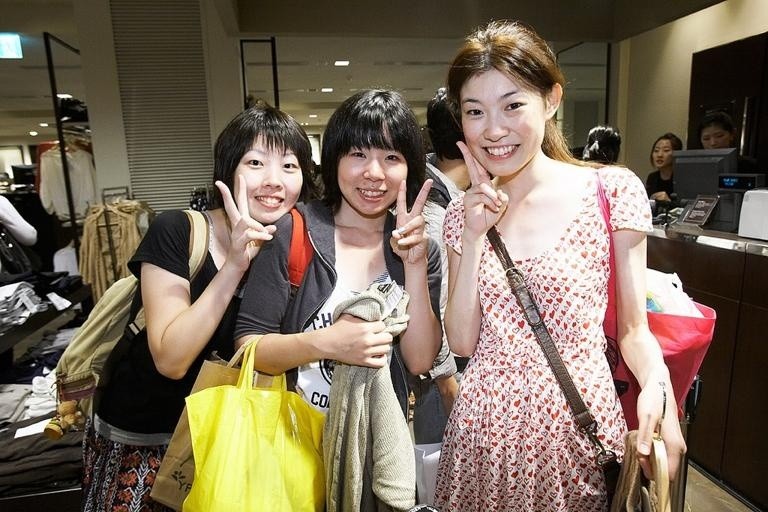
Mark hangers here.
[84,195,146,231]
[40,137,94,157]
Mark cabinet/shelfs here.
[647,228,768,512]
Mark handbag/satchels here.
[180,336,334,512]
[612,431,675,512]
[589,172,715,441]
[148,339,277,512]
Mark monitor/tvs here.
[672,148,741,231]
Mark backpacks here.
[53,207,209,432]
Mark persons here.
[643,133,687,214]
[442,24,688,511]
[0,195,37,247]
[236,89,445,512]
[699,113,737,162]
[581,125,623,164]
[412,87,492,442]
[83,98,316,511]
